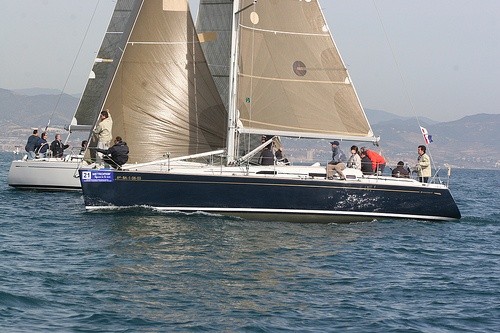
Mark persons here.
[392,161,410,177]
[325,141,348,180]
[24,110,130,169]
[259,134,289,173]
[347,145,385,176]
[415,146,432,184]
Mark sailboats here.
[6,0,229,191]
[73,0,463,222]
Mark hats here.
[330,141,339,145]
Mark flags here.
[422,126,433,145]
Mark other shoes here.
[323,176,334,180]
[338,178,346,181]
